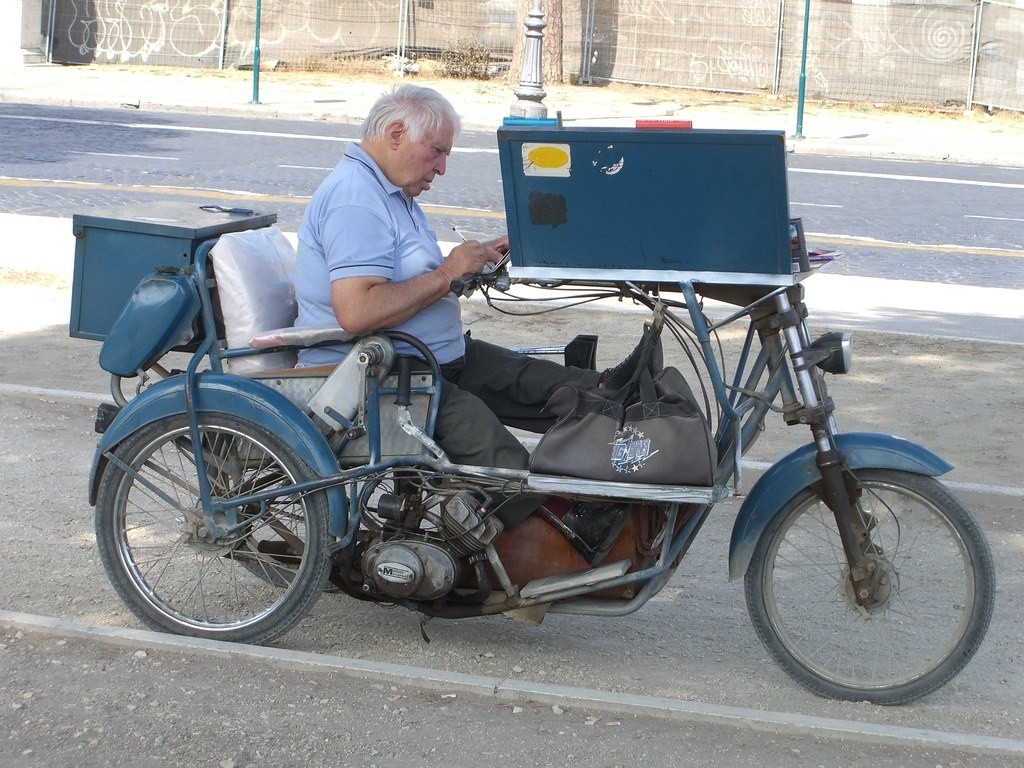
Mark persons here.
[292,83,663,568]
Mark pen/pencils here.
[452,226,493,269]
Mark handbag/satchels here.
[528,336,718,486]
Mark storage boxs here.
[67,205,279,352]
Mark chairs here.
[199,224,441,462]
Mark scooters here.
[67,110,995,705]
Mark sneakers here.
[561,501,630,568]
[604,330,663,389]
